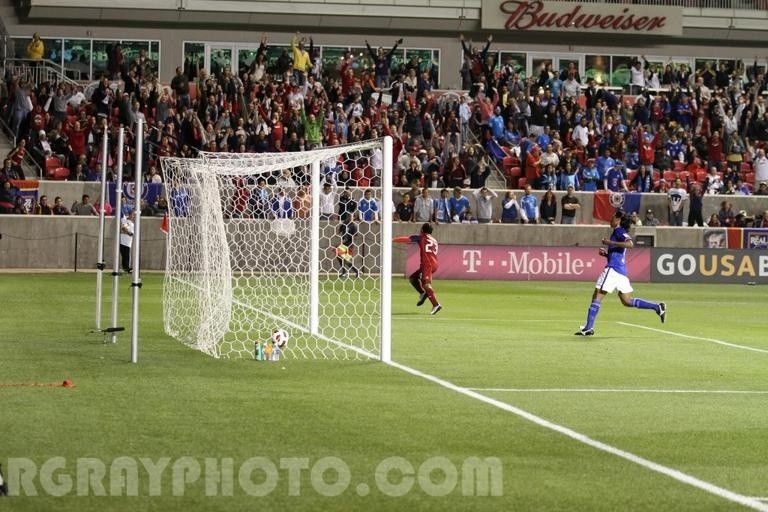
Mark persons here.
[0,25,768,235]
[568,210,668,335]
[115,208,139,273]
[333,212,365,278]
[390,221,444,315]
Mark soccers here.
[270,329,287,347]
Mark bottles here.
[254,340,280,363]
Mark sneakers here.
[416,292,428,306]
[574,328,594,335]
[657,302,666,323]
[431,304,441,314]
